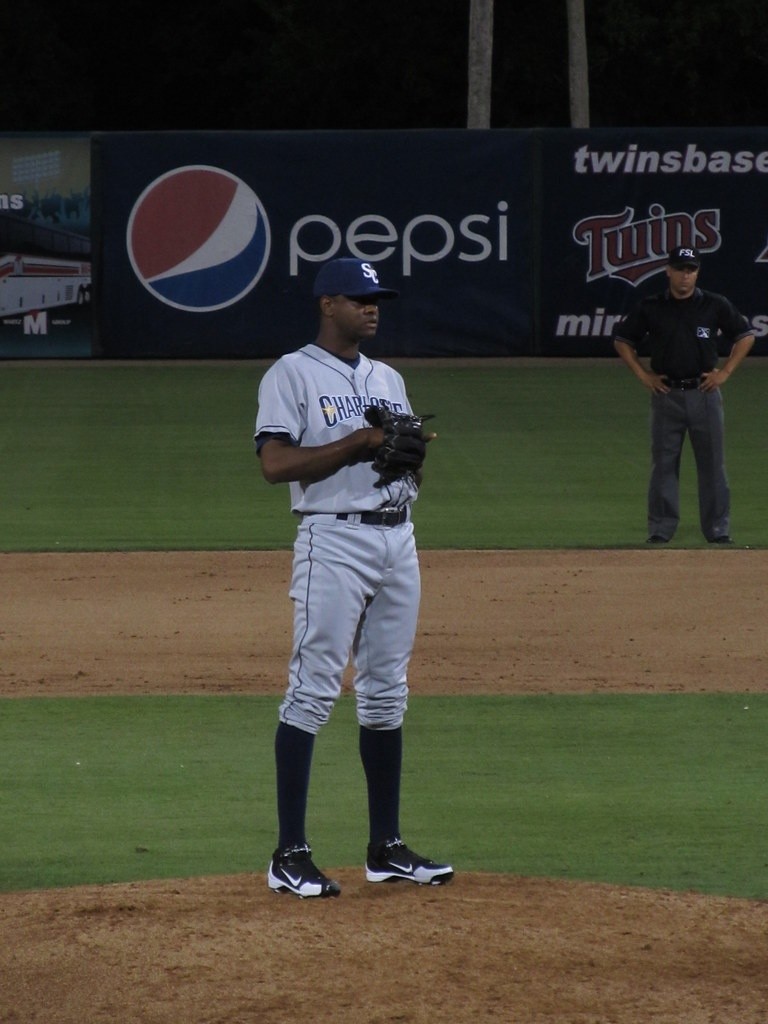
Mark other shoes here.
[708,536,734,544]
[646,536,668,544]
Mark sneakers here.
[268,843,340,898]
[365,838,454,886]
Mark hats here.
[669,246,701,268]
[313,257,400,298]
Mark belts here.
[662,378,704,388]
[336,505,407,525]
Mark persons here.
[615,245,755,545]
[254,259,455,899]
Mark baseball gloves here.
[362,402,430,489]
